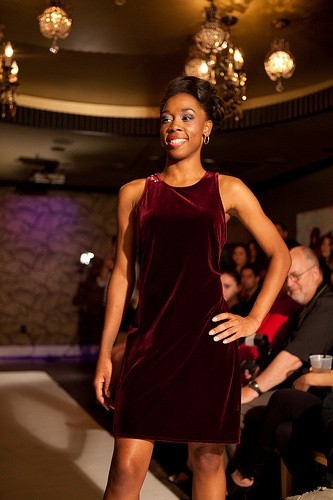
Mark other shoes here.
[168,473,189,484]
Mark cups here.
[309,354,333,373]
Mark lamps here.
[185,0,296,110]
[37,0,72,55]
[0,22,18,119]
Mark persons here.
[94,220,333,500]
[91,77,293,500]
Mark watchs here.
[248,379,262,394]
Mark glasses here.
[287,265,315,283]
